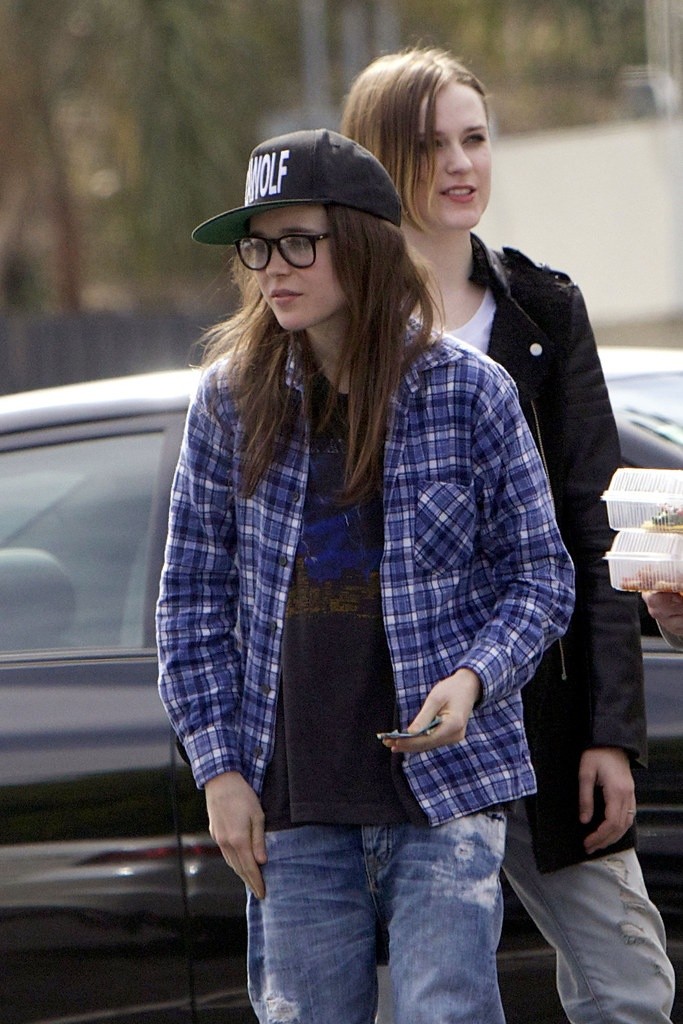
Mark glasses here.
[235,232,332,270]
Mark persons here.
[154,128,577,1024]
[339,32,675,1024]
[641,505,683,652]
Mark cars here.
[0,345,683,1024]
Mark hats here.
[191,130,402,245]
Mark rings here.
[628,809,636,816]
